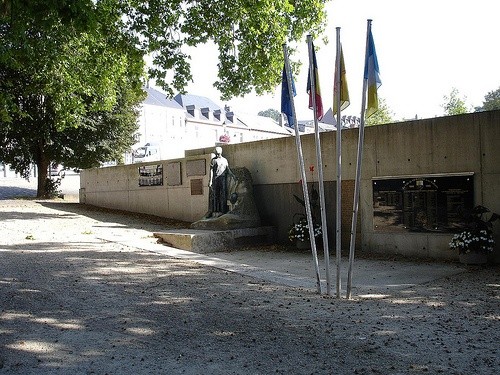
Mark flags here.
[279,51,297,128]
[332,35,351,118]
[364,27,383,119]
[305,40,324,122]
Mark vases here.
[296,240,312,249]
[460,252,487,263]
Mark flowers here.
[447,230,496,254]
[288,220,323,241]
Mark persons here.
[204,147,236,219]
